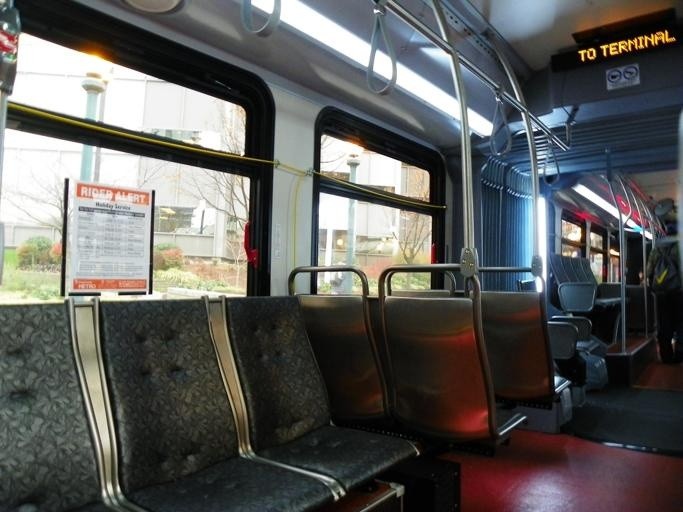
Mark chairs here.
[288,264,390,423]
[375,264,526,448]
[215,297,435,499]
[85,294,345,511]
[0,288,119,512]
[385,258,654,408]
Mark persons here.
[644,211,682,365]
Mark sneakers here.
[660,355,683,364]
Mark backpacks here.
[651,239,681,291]
